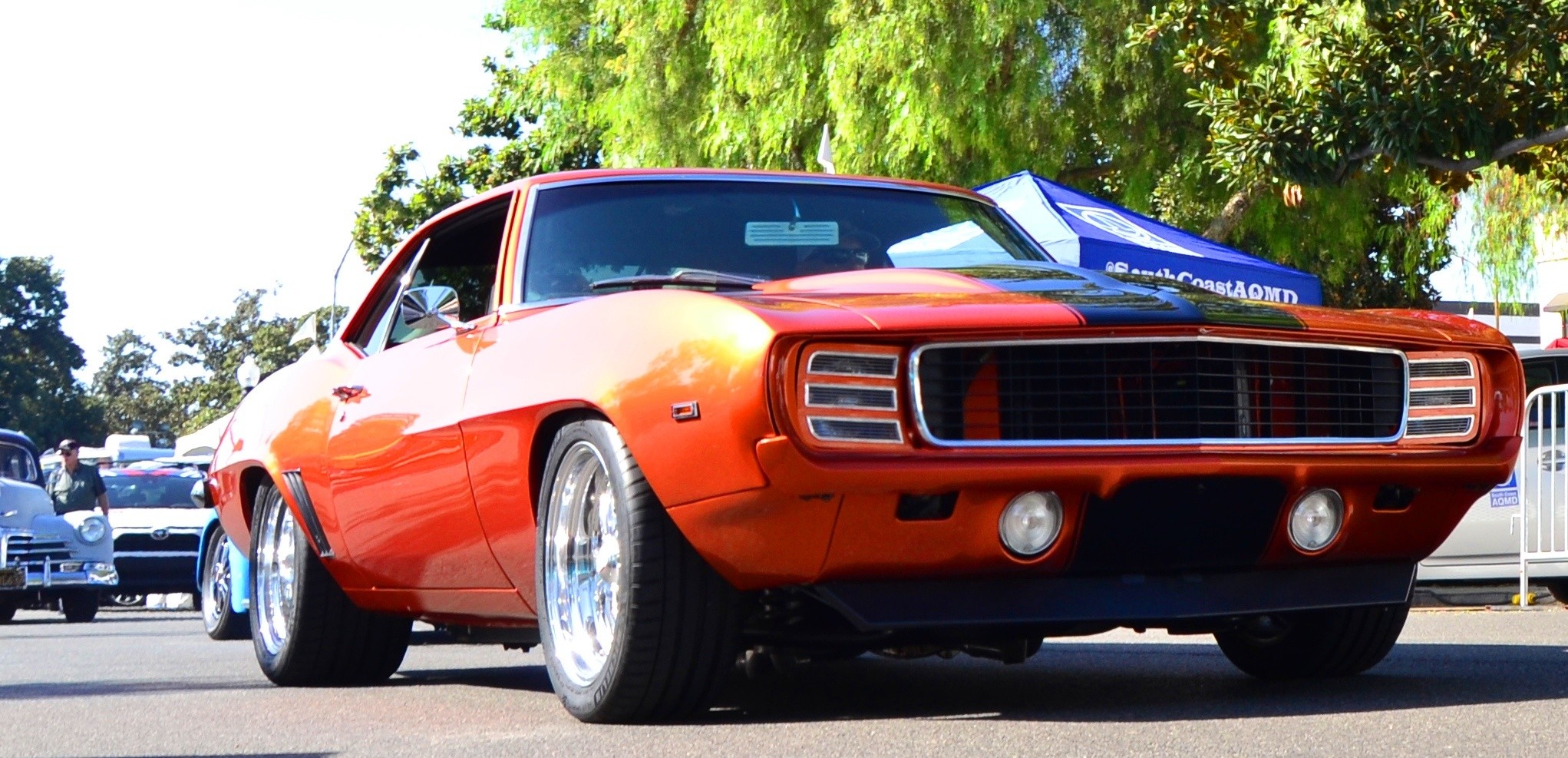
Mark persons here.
[45,438,108,521]
[787,220,883,280]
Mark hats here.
[56,439,80,451]
[797,219,881,263]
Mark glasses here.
[807,249,869,266]
[61,452,71,457]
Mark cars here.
[1417,349,1568,605]
[1,429,257,639]
[205,165,1530,729]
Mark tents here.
[886,167,1324,310]
